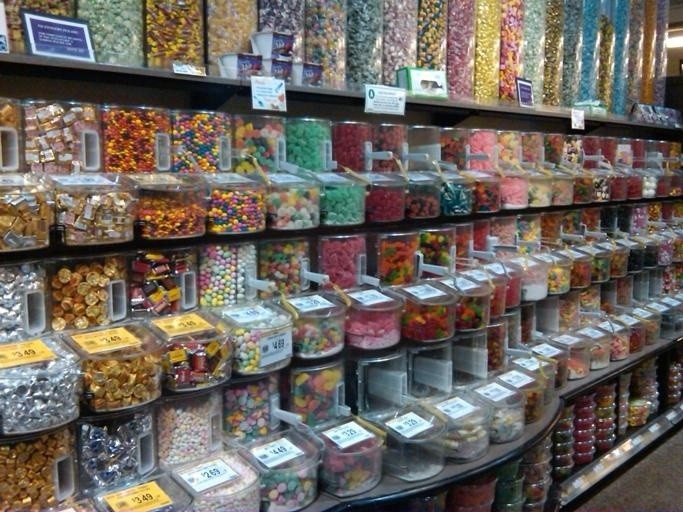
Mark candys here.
[0,0,682,512]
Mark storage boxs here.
[0,96,683,507]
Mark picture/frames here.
[17,6,98,65]
[0,48,681,510]
